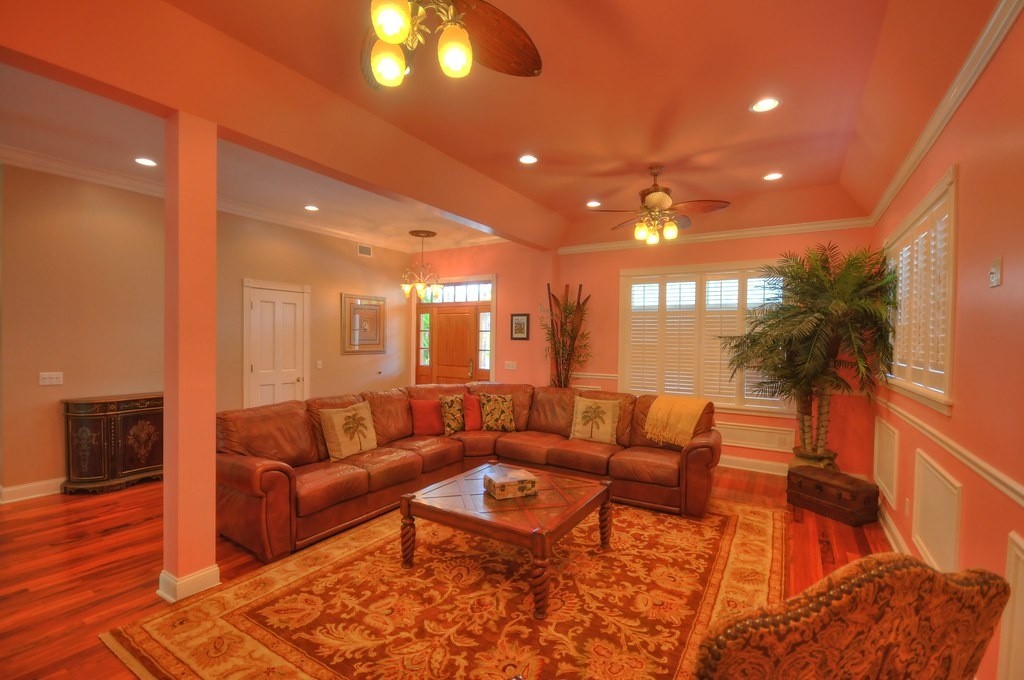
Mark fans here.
[587,165,731,230]
[360,0,542,90]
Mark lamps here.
[634,192,680,245]
[370,0,473,86]
[400,230,445,300]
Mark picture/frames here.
[511,313,529,339]
[341,293,387,354]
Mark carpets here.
[97,497,792,680]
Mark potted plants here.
[711,241,899,468]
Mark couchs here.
[216,376,722,564]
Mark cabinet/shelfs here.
[60,391,164,494]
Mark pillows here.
[440,395,465,436]
[569,395,620,445]
[463,392,482,431]
[409,397,445,435]
[478,392,516,432]
[319,400,377,463]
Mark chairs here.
[692,552,1011,680]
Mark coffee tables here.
[399,459,613,620]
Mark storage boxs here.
[483,469,539,500]
[786,466,880,527]
